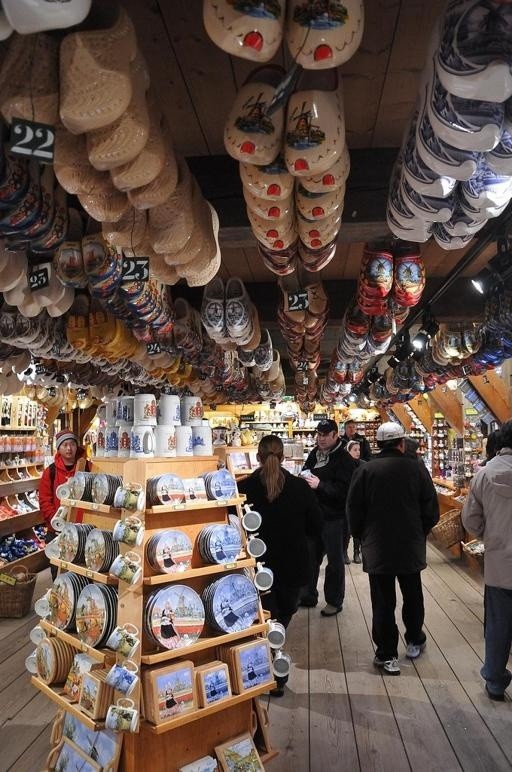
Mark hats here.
[375,421,407,441]
[315,419,337,433]
[56,430,81,450]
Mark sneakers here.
[321,604,342,616]
[405,640,426,658]
[375,656,401,676]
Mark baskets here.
[431,509,465,549]
[460,538,484,578]
[0,565,38,618]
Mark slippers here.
[1,1,284,410]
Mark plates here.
[146,470,237,507]
[59,522,119,574]
[144,525,242,576]
[144,575,258,651]
[38,636,80,686]
[73,472,123,506]
[52,573,119,651]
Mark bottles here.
[295,434,313,447]
[432,420,448,473]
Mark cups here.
[96,393,213,456]
[44,535,61,560]
[50,507,69,530]
[103,697,141,734]
[25,647,39,673]
[112,518,142,544]
[108,621,140,659]
[243,504,274,591]
[112,482,145,512]
[108,552,143,585]
[267,619,292,678]
[30,618,47,646]
[56,483,71,500]
[104,659,140,697]
[35,588,53,616]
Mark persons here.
[346,422,440,676]
[297,419,358,617]
[340,420,369,460]
[235,435,323,697]
[39,431,89,582]
[485,429,499,460]
[343,440,368,564]
[461,418,512,701]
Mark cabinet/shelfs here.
[30,453,280,771]
[248,419,486,487]
[0,392,57,579]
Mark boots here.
[343,537,361,563]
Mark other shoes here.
[387,0,512,250]
[428,319,512,391]
[277,277,329,413]
[320,242,428,407]
[284,0,364,277]
